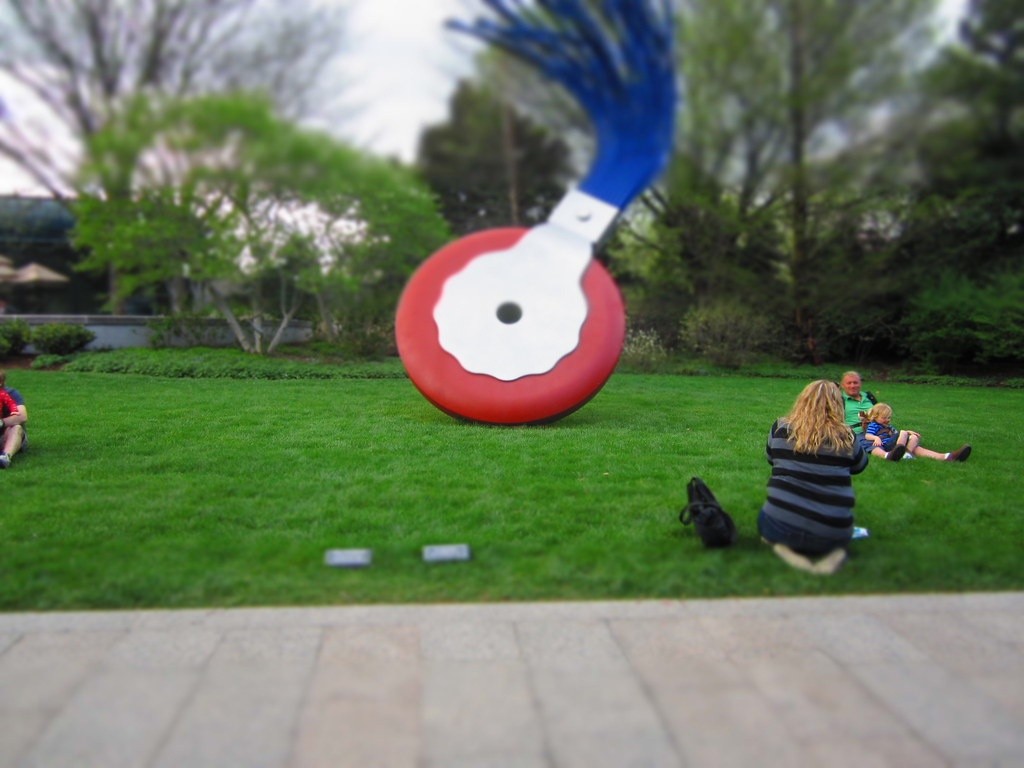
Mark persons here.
[0,390,20,436]
[757,380,868,575]
[865,402,920,459]
[0,371,27,469]
[838,371,972,462]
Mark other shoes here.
[902,453,912,459]
[0,454,9,468]
[886,444,906,461]
[774,543,811,570]
[814,547,846,575]
[945,444,972,462]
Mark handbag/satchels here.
[678,476,740,547]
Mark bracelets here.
[2,419,5,427]
